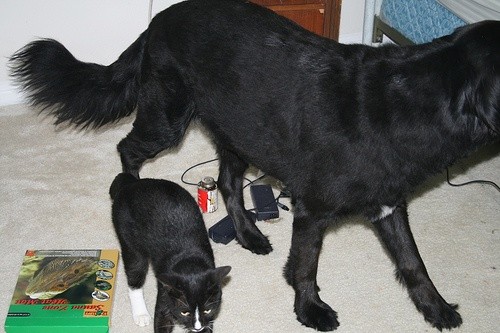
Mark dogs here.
[2,1,498,332]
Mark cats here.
[108,172,232,332]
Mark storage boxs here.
[5,249,119,333]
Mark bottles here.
[198,177,218,213]
